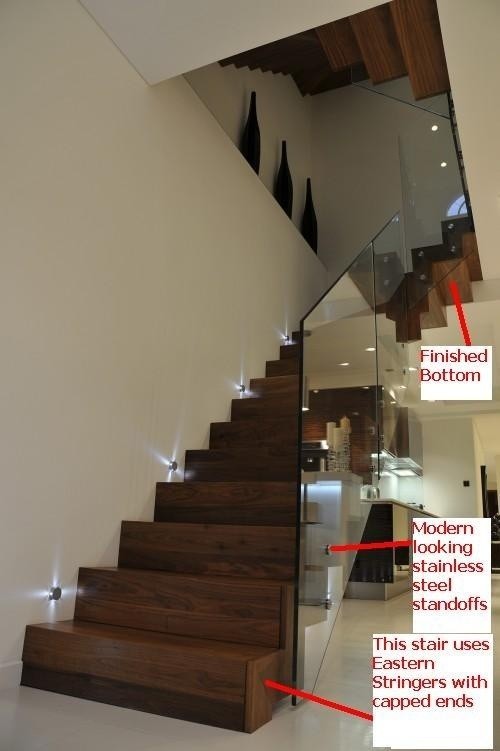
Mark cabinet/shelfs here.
[302,385,424,486]
